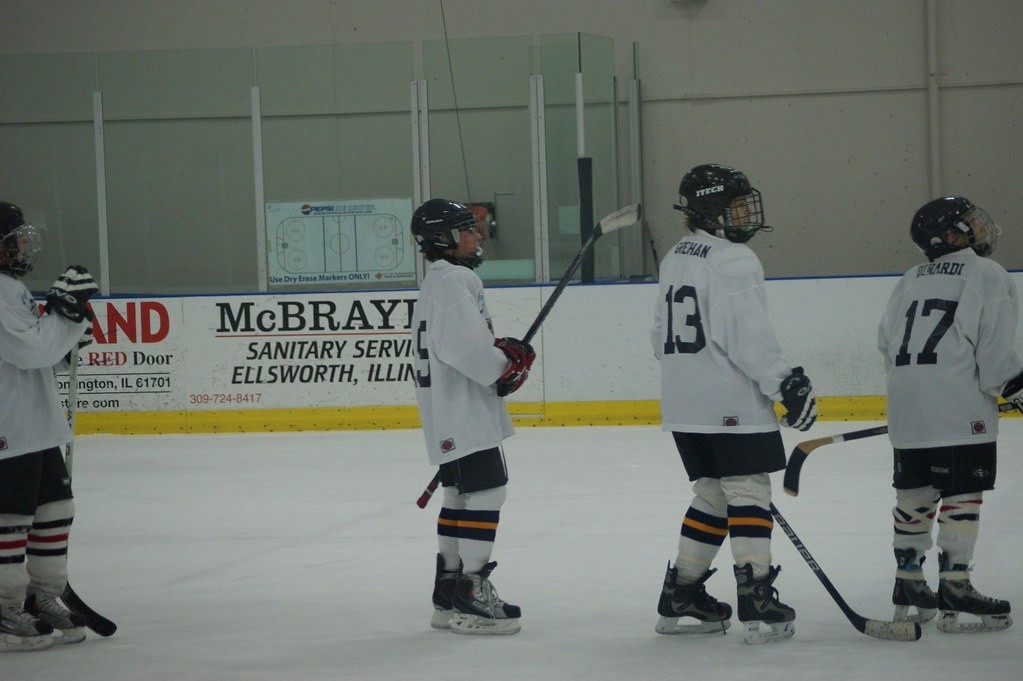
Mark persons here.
[0,202,99,653]
[411,198,536,635]
[651,164,818,643]
[878,196,1023,633]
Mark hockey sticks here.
[65,343,118,637]
[771,501,923,642]
[416,203,642,509]
[783,401,1018,496]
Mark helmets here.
[411,199,485,270]
[674,163,773,244]
[0,201,38,277]
[910,196,996,257]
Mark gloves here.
[493,336,536,398]
[44,265,99,323]
[781,366,818,432]
[1001,372,1023,413]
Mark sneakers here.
[892,547,939,624]
[937,551,1012,633]
[733,563,795,646]
[0,598,54,651]
[430,553,463,628]
[655,560,732,634]
[450,558,521,635]
[24,588,88,645]
